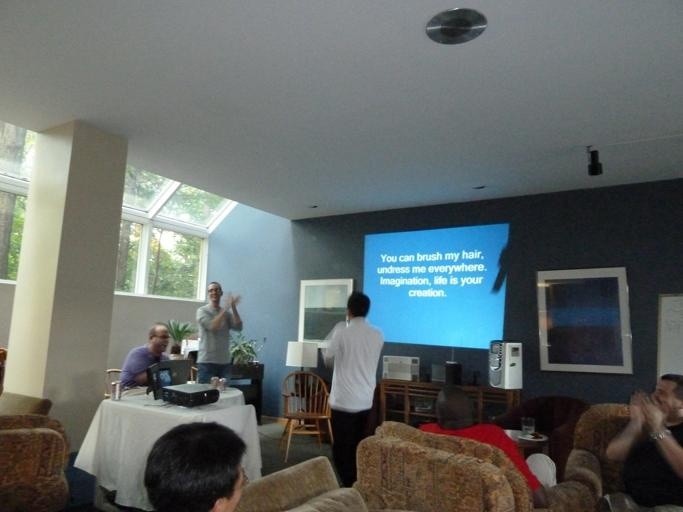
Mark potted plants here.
[164,319,198,354]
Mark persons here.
[321,289,385,488]
[196,281,243,384]
[142,421,249,512]
[416,384,558,507]
[117,322,173,397]
[605,373,682,511]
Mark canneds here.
[210,376,219,391]
[219,377,228,394]
[110,380,121,402]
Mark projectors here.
[161,382,220,406]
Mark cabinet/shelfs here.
[378,379,521,426]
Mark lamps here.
[588,151,604,175]
[285,341,319,372]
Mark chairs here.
[277,370,334,464]
[0,347,73,512]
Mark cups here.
[522,416,536,435]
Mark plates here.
[518,434,549,442]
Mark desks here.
[73,393,264,511]
[120,383,246,405]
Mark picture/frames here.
[536,266,633,375]
[298,278,355,349]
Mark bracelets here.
[650,430,670,441]
